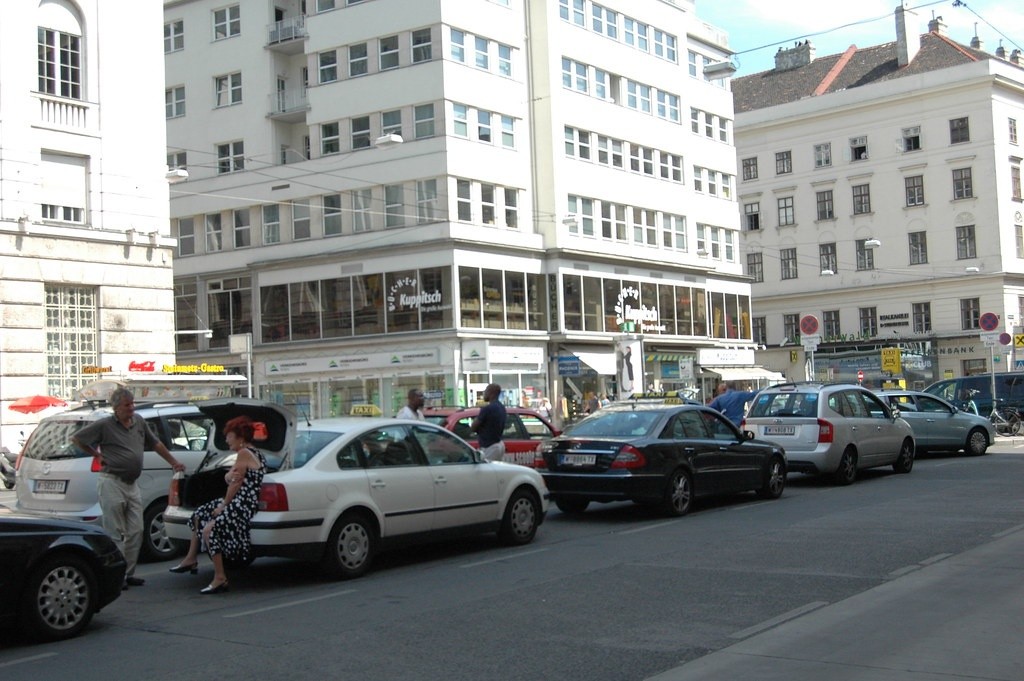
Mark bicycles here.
[960,389,1021,438]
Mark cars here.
[863,388,996,456]
[534,401,789,516]
[0,511,127,650]
[162,417,551,580]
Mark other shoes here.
[126,577,145,585]
[121,586,128,591]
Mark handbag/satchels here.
[169,471,186,506]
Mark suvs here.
[739,381,916,485]
[15,400,216,561]
[420,405,560,476]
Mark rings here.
[232,479,235,482]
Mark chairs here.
[169,422,188,450]
[385,444,414,464]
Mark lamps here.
[564,215,581,227]
[166,167,190,183]
[820,268,834,276]
[866,240,881,249]
[696,249,711,256]
[17,216,32,236]
[378,133,403,148]
[967,267,979,274]
[147,231,163,246]
[128,228,141,245]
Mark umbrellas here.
[8,396,68,421]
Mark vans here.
[918,371,1024,424]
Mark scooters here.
[0,430,28,489]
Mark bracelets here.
[222,500,228,506]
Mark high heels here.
[169,562,198,574]
[201,578,229,594]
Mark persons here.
[658,383,665,392]
[704,381,766,434]
[471,384,506,461]
[397,389,425,421]
[615,343,635,392]
[170,416,268,594]
[72,388,185,590]
[584,391,610,414]
[647,383,655,392]
[537,398,552,440]
